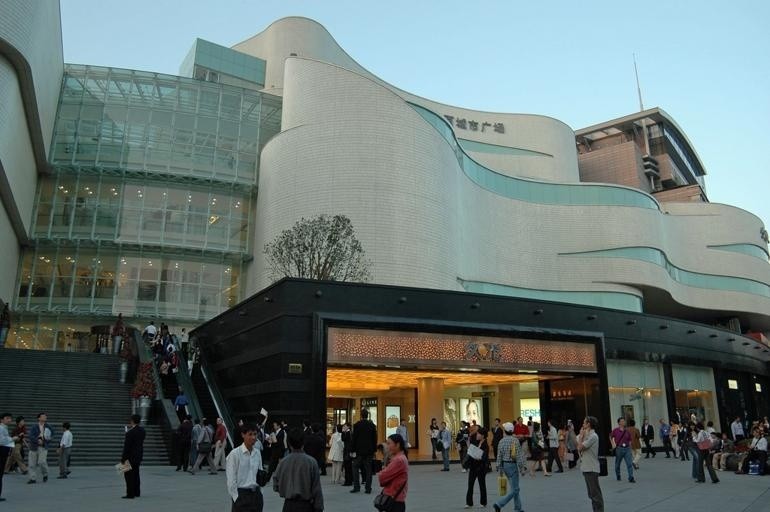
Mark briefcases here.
[496,471,509,496]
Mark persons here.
[4,416,28,474]
[145,321,195,421]
[492,423,526,512]
[396,418,410,455]
[174,416,244,476]
[56,422,73,479]
[225,419,325,512]
[0,413,19,501]
[121,415,146,499]
[430,417,579,477]
[576,415,605,512]
[463,427,491,508]
[611,416,770,483]
[27,413,52,483]
[328,408,389,494]
[377,434,409,512]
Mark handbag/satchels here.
[695,438,714,451]
[256,468,269,487]
[372,493,394,512]
[467,442,484,462]
[597,457,609,477]
[607,447,616,457]
[43,428,52,441]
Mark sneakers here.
[0,465,144,501]
[331,478,372,494]
[440,467,468,473]
[175,463,228,475]
[464,502,502,512]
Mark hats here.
[502,421,515,433]
